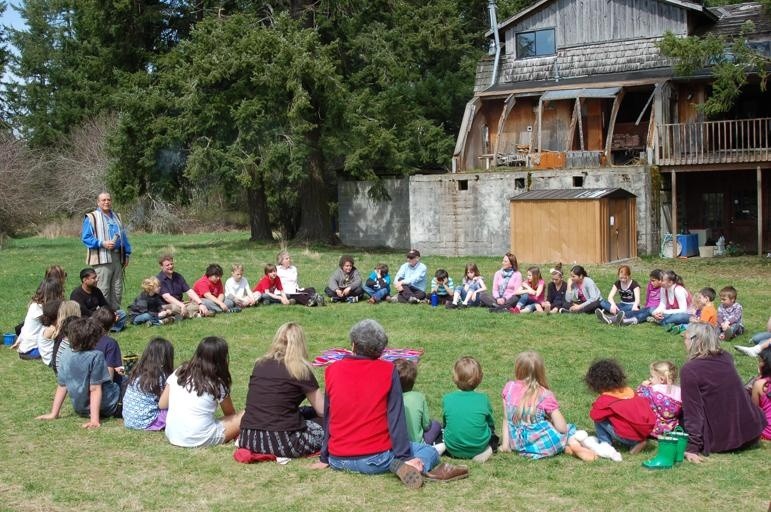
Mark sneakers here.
[595,308,611,325]
[647,316,661,324]
[208,310,215,317]
[733,344,761,358]
[496,304,510,313]
[514,306,520,314]
[330,297,340,302]
[368,298,375,304]
[230,307,241,313]
[559,308,569,313]
[345,296,358,303]
[289,299,296,305]
[446,304,458,309]
[308,292,318,307]
[665,323,674,331]
[146,320,152,328]
[161,316,175,324]
[317,295,324,306]
[386,295,398,303]
[456,305,467,310]
[616,311,625,327]
[409,297,420,304]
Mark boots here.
[669,426,689,464]
[642,432,678,469]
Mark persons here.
[309,319,424,490]
[594,269,664,326]
[559,266,603,314]
[600,266,641,315]
[239,321,324,458]
[121,337,174,431]
[70,268,126,333]
[751,346,771,440]
[636,360,683,439]
[735,318,771,358]
[445,263,488,309]
[362,263,391,304]
[34,318,111,429]
[252,264,296,305]
[324,255,364,304]
[391,249,427,304]
[509,266,545,314]
[37,301,61,367]
[81,191,132,310]
[679,322,768,463]
[155,255,209,319]
[534,270,567,315]
[91,305,122,383]
[646,270,694,324]
[586,361,656,455]
[393,357,441,446]
[480,252,523,312]
[56,315,80,372]
[274,250,324,306]
[158,337,244,448]
[51,301,81,375]
[127,276,176,328]
[224,263,261,307]
[426,269,454,305]
[16,278,65,359]
[715,286,744,339]
[9,265,68,349]
[433,357,499,462]
[689,287,717,327]
[498,350,597,462]
[190,264,242,317]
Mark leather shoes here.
[388,459,424,489]
[423,462,468,483]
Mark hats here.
[406,249,420,259]
